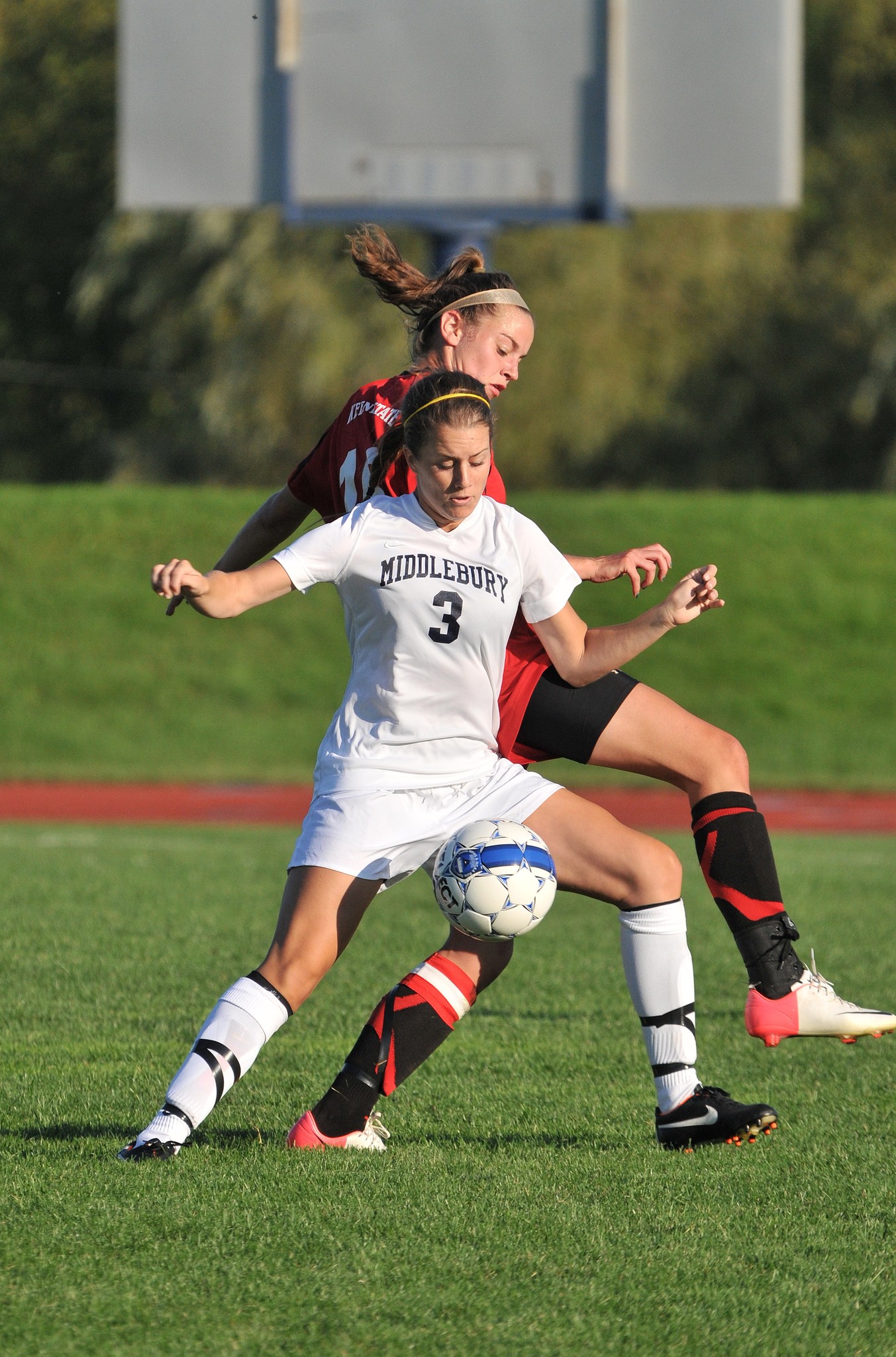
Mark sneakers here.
[745,948,896,1048]
[655,1082,779,1154]
[116,1135,192,1163]
[286,1109,390,1150]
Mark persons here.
[162,220,896,1152]
[118,369,777,1163]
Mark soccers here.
[431,817,558,945]
